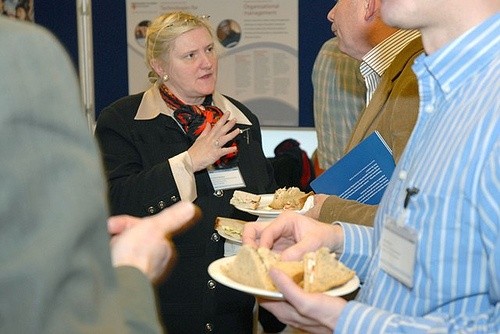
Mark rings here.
[215,139,220,148]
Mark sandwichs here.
[221,248,355,293]
[267,187,313,210]
[230,191,261,210]
[214,217,247,240]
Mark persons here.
[300,0,420,226]
[312,37,367,172]
[94,12,278,334]
[0,17,195,334]
[241,0,500,334]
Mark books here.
[310,131,394,206]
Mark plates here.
[236,193,313,216]
[207,255,360,300]
